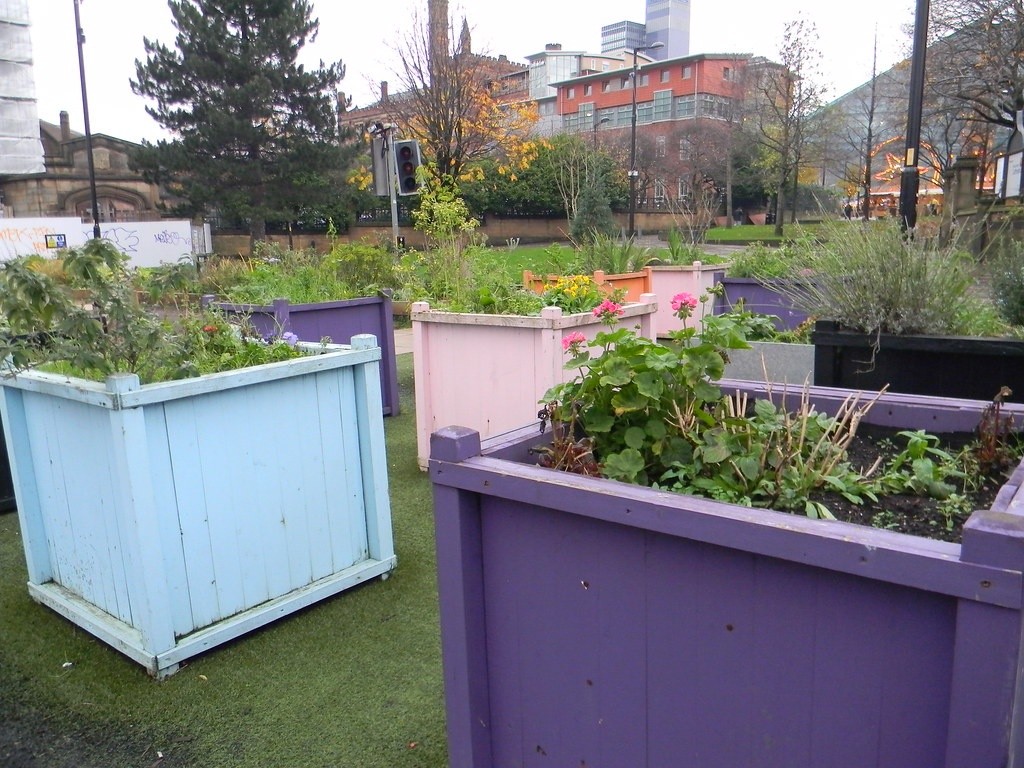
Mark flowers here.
[542,274,603,313]
[389,242,430,298]
[526,294,894,524]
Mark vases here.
[391,299,454,315]
[428,377,1024,768]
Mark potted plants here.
[409,167,658,473]
[645,225,734,338]
[202,217,401,417]
[750,188,1024,404]
[0,235,397,683]
[523,222,651,303]
[714,234,869,332]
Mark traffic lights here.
[393,139,423,196]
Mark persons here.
[733,207,743,227]
[845,204,852,220]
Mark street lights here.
[594,117,610,146]
[628,41,665,243]
